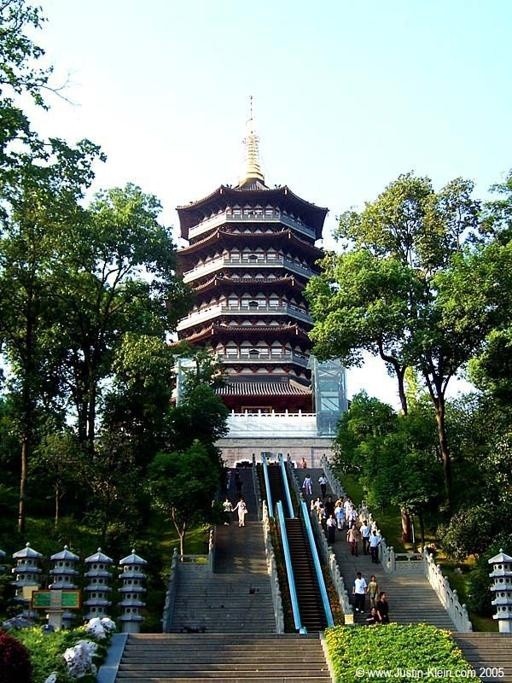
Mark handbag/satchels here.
[301,485,305,488]
[244,508,248,513]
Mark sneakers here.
[355,607,365,614]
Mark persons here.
[234,469,241,482]
[351,572,367,614]
[301,457,307,468]
[225,468,232,490]
[223,496,234,526]
[233,497,247,528]
[234,478,243,495]
[377,591,390,625]
[302,473,381,564]
[365,607,383,625]
[367,574,380,608]
